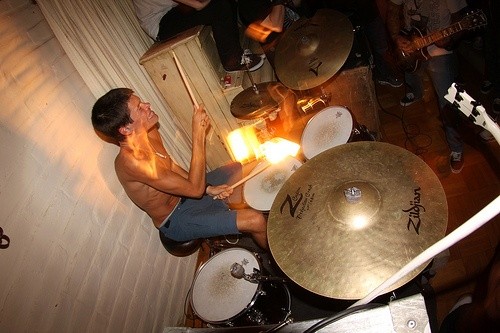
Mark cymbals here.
[273,9,354,90]
[230,81,288,120]
[267,141,448,300]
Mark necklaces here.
[139,139,166,159]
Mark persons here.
[438,254,500,333]
[387,0,469,175]
[91,87,269,254]
[132,0,403,87]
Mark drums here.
[189,247,291,329]
[242,155,307,214]
[299,105,376,160]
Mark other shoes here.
[379,74,403,87]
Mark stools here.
[158,228,227,272]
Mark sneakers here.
[450,151,463,173]
[224,52,267,73]
[400,91,422,107]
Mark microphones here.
[231,262,245,278]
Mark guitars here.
[380,8,488,87]
[444,82,500,147]
